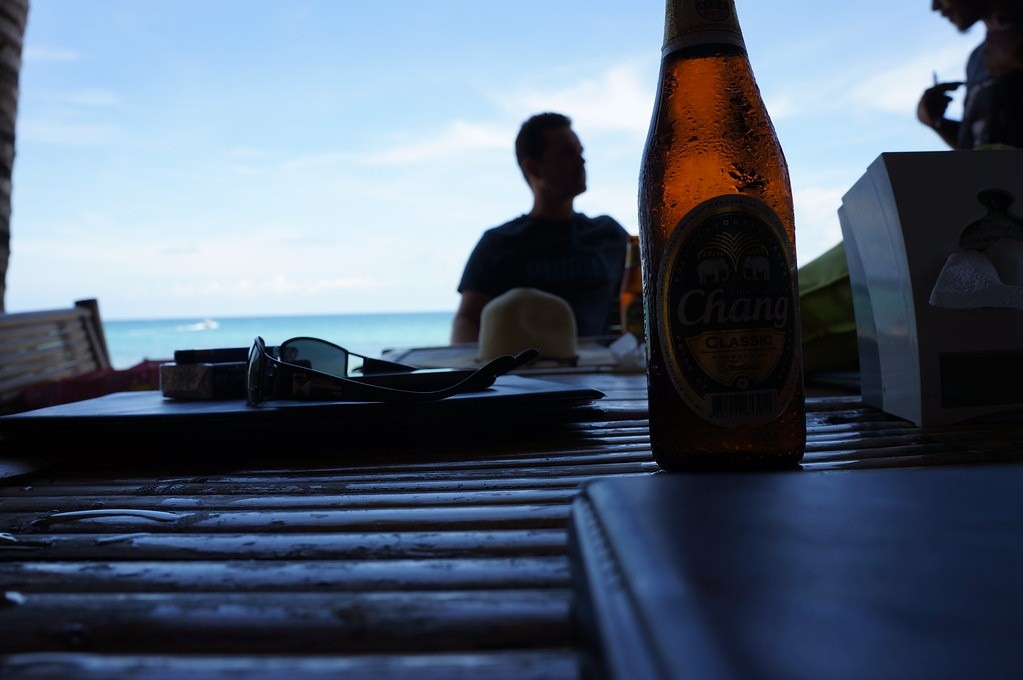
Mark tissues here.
[837,147,1023,427]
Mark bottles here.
[635,0,808,475]
[620,235,646,345]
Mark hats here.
[480,288,579,368]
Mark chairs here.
[0,298,114,385]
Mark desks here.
[0,372,1023,680]
[393,338,616,371]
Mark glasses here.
[245,336,541,401]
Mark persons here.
[916,0,1023,148]
[450,113,631,345]
